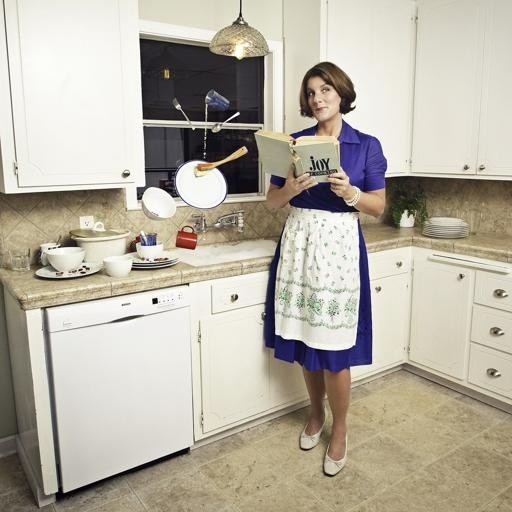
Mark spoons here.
[211,112,240,133]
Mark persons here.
[263,61,386,478]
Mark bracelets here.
[344,185,361,207]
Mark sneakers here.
[324,427,348,475]
[300,407,328,449]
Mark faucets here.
[214,210,244,233]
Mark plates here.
[35,262,102,279]
[174,159,227,210]
[123,251,179,269]
[421,217,469,240]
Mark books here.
[253,129,340,183]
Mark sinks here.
[166,239,277,267]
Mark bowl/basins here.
[46,247,85,272]
[136,241,164,260]
[102,256,133,278]
[142,187,177,221]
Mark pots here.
[70,222,131,268]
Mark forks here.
[172,98,195,131]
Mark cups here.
[39,243,62,266]
[176,226,198,250]
[205,89,230,113]
[8,247,31,271]
[140,234,157,246]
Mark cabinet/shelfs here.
[348,247,413,382]
[409,1,512,183]
[190,269,309,442]
[408,246,512,406]
[210,1,271,61]
[0,0,146,195]
